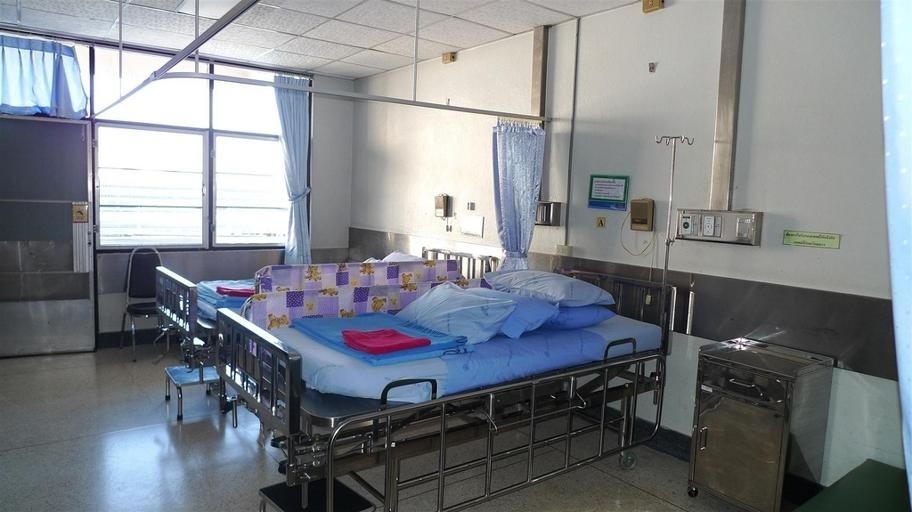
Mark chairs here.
[121,247,170,361]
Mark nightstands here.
[689,337,834,512]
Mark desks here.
[795,459,909,511]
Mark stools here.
[164,367,220,419]
[260,478,376,512]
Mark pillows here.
[554,305,617,331]
[362,258,382,263]
[483,270,616,308]
[466,286,557,339]
[382,250,425,262]
[394,282,517,345]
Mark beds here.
[156,247,500,368]
[215,270,673,512]
